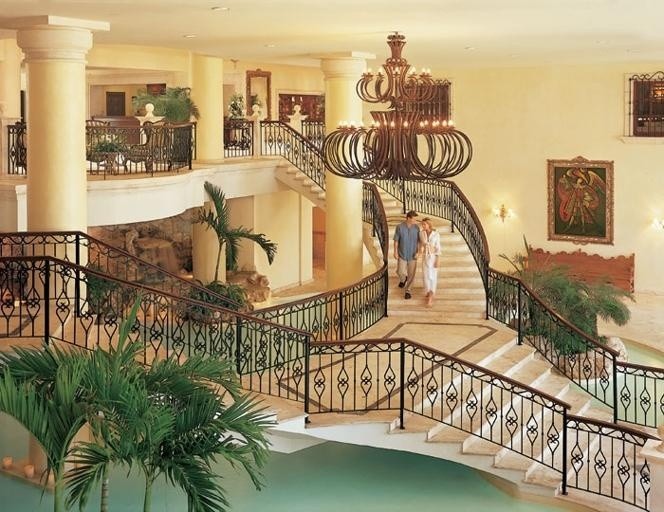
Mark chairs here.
[16,119,191,176]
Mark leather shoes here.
[405,293,410,299]
[400,277,407,287]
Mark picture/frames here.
[547,155,614,246]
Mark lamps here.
[496,205,511,221]
[321,32,473,180]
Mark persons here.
[417,217,441,309]
[393,210,421,300]
